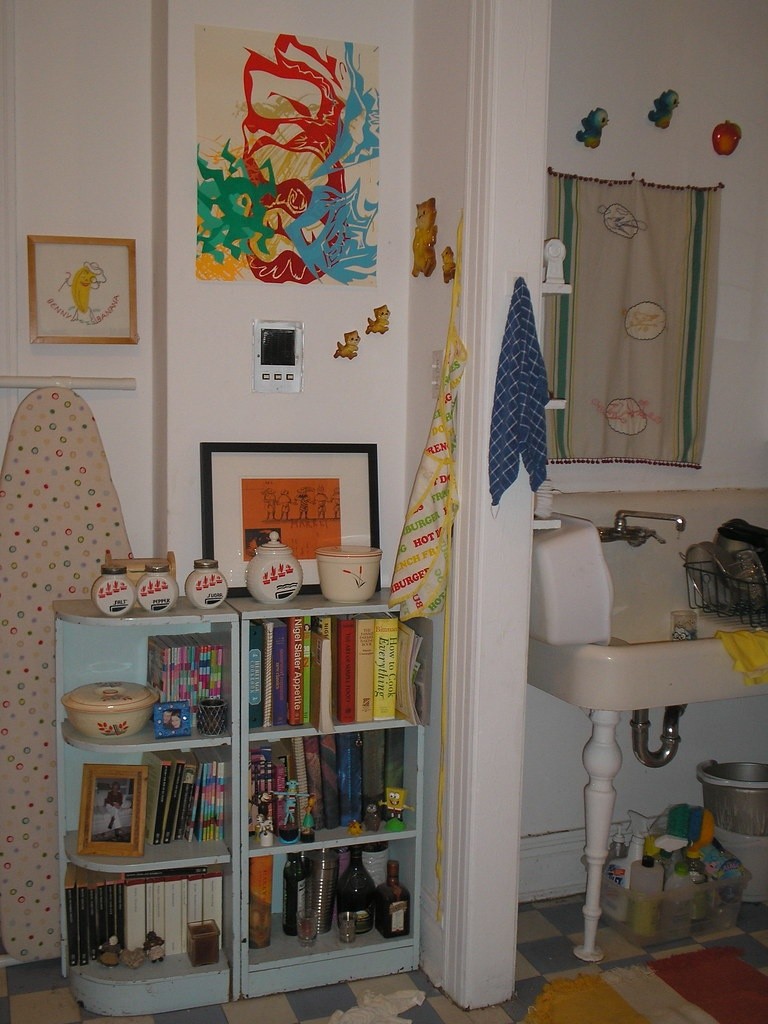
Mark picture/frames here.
[153,699,193,740]
[77,763,149,858]
[27,235,138,345]
[200,441,383,597]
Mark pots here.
[679,541,745,613]
[695,759,768,836]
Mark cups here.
[296,908,318,948]
[337,912,358,944]
[196,698,228,736]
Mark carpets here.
[520,949,768,1024]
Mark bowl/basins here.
[61,681,160,739]
[315,545,383,605]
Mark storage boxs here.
[187,919,220,967]
[580,854,751,946]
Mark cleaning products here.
[600,803,746,940]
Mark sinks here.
[557,603,742,665]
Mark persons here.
[163,711,181,728]
[105,782,122,836]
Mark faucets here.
[595,510,688,547]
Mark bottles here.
[375,860,411,938]
[282,851,306,936]
[136,561,178,614]
[244,531,304,605]
[629,839,744,938]
[304,849,340,935]
[184,558,228,609]
[337,845,377,933]
[90,563,136,617]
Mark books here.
[249,612,398,728]
[142,747,224,844]
[249,727,405,838]
[65,862,222,966]
[147,632,223,712]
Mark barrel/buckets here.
[713,826,768,902]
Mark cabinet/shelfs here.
[51,588,433,1017]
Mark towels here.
[487,275,549,520]
